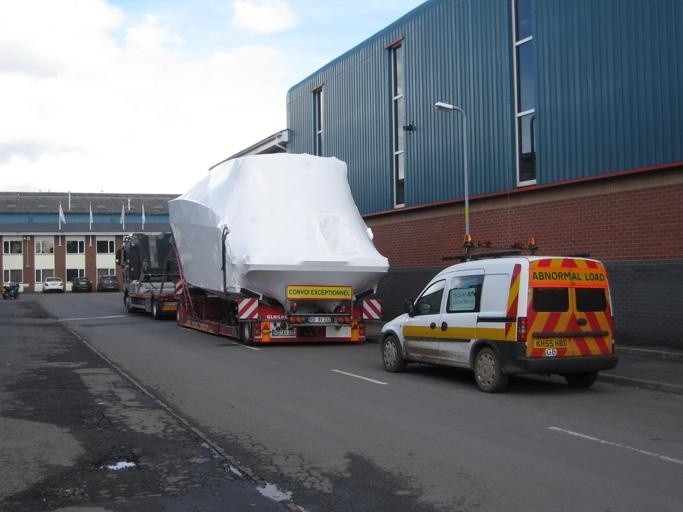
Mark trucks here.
[115,233,390,343]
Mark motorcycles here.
[2,284,19,300]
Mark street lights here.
[434,102,469,261]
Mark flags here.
[58,204,66,225]
[140,203,146,224]
[88,204,93,225]
[118,204,124,225]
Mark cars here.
[43,276,119,293]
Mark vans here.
[380,255,617,393]
